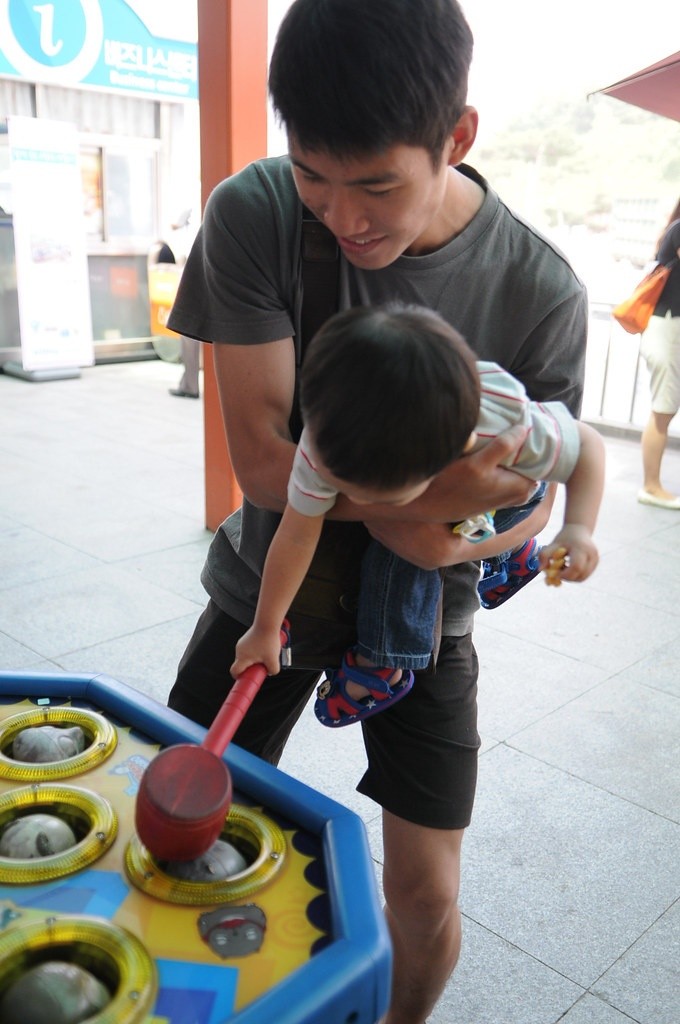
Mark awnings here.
[586,50,680,123]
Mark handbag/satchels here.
[613,261,675,334]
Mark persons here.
[638,198,680,511]
[165,0,591,1024]
[230,303,606,725]
[169,336,200,398]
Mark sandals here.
[314,644,415,728]
[479,538,545,608]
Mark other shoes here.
[170,386,199,398]
[638,487,679,509]
